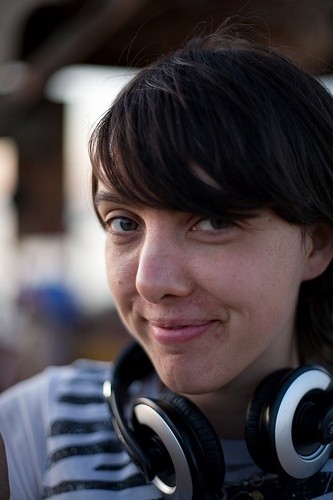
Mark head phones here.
[104,330,333,500]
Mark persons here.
[0,36,333,500]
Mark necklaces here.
[220,468,269,486]
